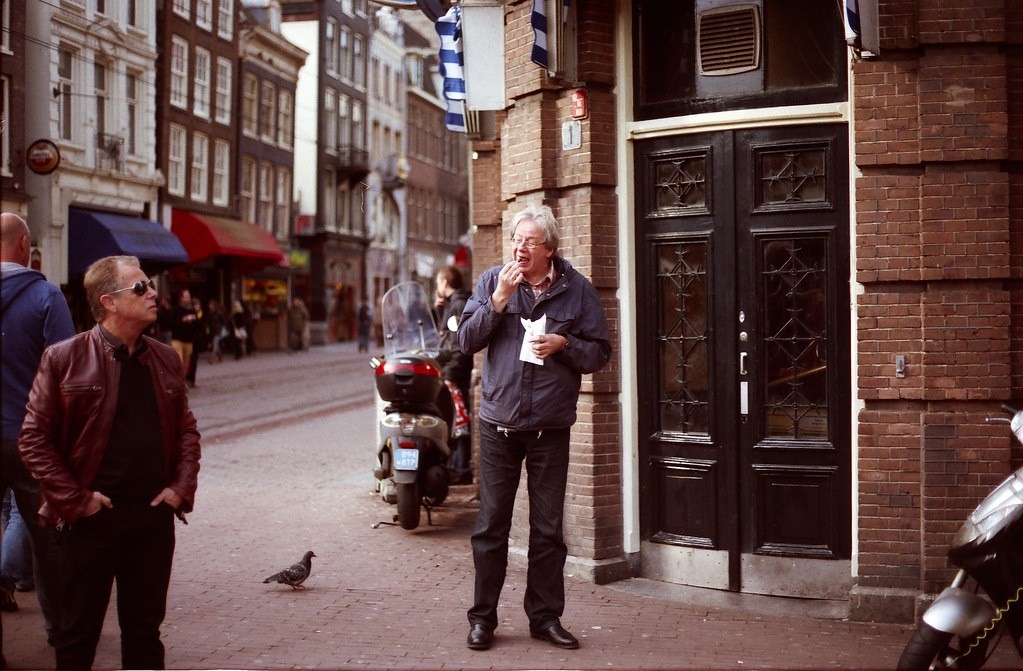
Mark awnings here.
[67,206,188,278]
[171,210,284,274]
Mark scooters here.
[368,282,473,533]
[893,403,1023,671]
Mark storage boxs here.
[374,357,443,402]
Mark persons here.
[330,279,373,353]
[288,299,309,355]
[0,212,77,612]
[432,265,473,487]
[19,255,202,671]
[205,299,250,366]
[456,204,612,650]
[168,287,202,389]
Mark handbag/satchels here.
[234,327,248,339]
[220,326,230,338]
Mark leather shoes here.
[529,624,579,649]
[467,623,495,649]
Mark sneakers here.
[15,580,35,591]
[0,575,18,612]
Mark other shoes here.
[206,356,214,364]
[450,472,474,486]
[217,355,223,363]
[186,379,195,388]
[45,627,55,646]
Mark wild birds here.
[262,551,317,591]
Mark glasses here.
[510,237,545,249]
[107,281,156,297]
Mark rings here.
[511,275,516,279]
